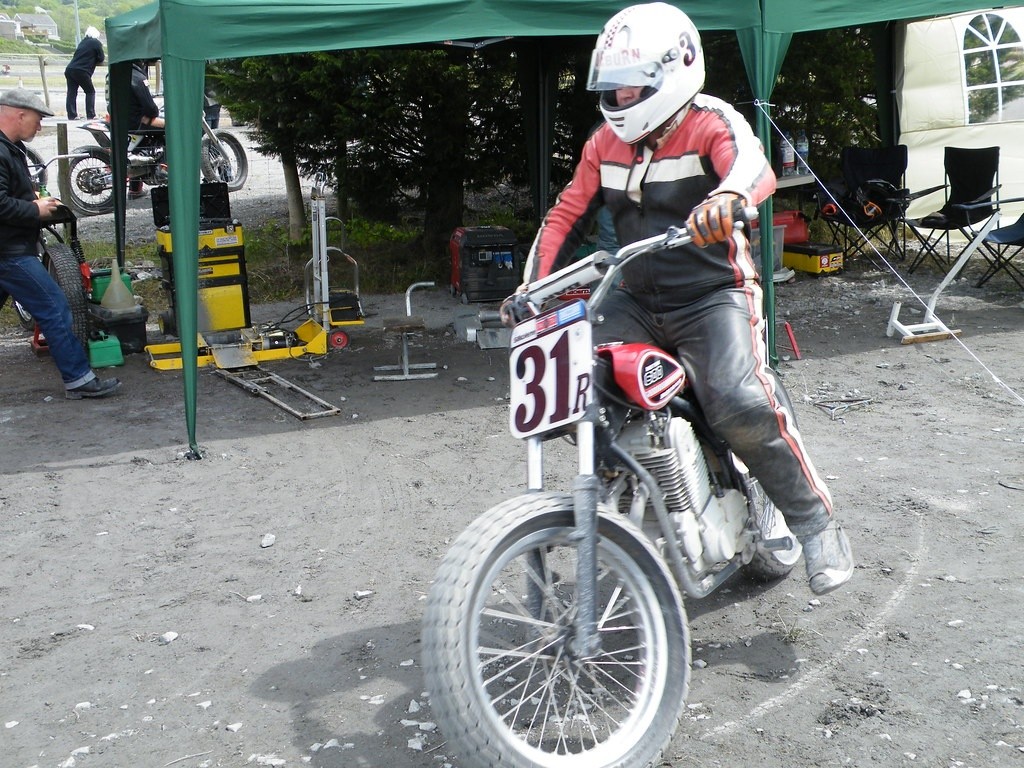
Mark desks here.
[775,173,815,189]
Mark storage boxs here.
[784,241,843,275]
[86,273,150,367]
[749,225,785,276]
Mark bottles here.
[796,130,808,175]
[39,185,50,199]
[780,131,795,176]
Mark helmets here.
[587,2,706,145]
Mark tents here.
[102,0,1024,459]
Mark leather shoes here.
[65,377,123,399]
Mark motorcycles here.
[10,143,91,353]
[55,91,250,216]
[418,206,805,767]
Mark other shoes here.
[93,116,103,120]
[160,166,168,177]
[75,115,81,119]
[128,186,148,200]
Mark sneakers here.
[803,512,855,596]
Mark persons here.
[63,28,240,200]
[498,2,856,594]
[0,87,123,400]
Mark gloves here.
[499,285,547,326]
[685,192,748,248]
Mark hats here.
[84,26,100,39]
[0,87,55,117]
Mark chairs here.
[820,144,1024,292]
